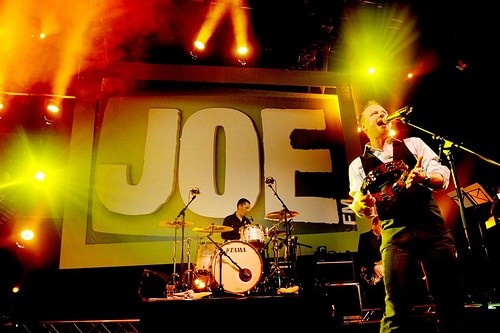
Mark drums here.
[209,239,264,295]
[238,222,267,252]
[194,241,223,271]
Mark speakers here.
[316,261,363,320]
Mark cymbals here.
[191,225,235,233]
[157,220,195,229]
[265,210,299,220]
[264,230,287,237]
[361,158,410,202]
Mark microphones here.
[382,106,413,124]
[266,179,274,183]
[191,188,201,194]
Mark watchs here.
[422,173,431,186]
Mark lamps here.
[237,46,249,65]
[44,98,62,125]
[15,229,34,249]
[190,41,206,58]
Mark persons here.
[221,198,271,277]
[349,100,467,333]
[358,216,386,309]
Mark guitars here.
[361,260,384,285]
